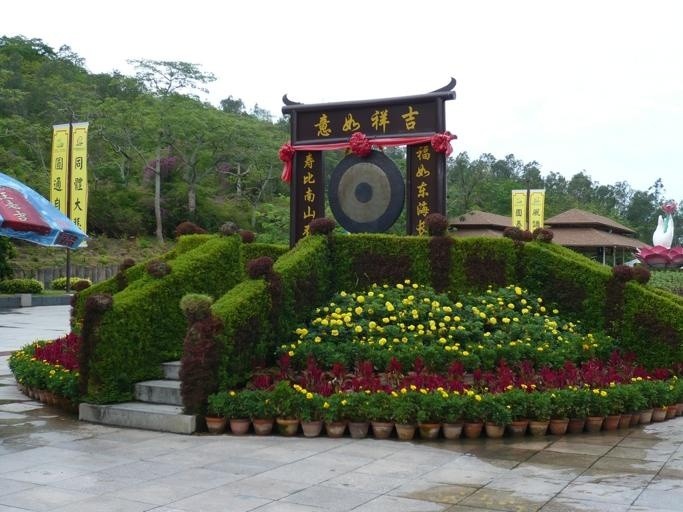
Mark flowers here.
[6,352,77,391]
[199,376,683,423]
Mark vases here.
[204,405,682,439]
[14,379,76,411]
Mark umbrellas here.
[0,158,88,249]
